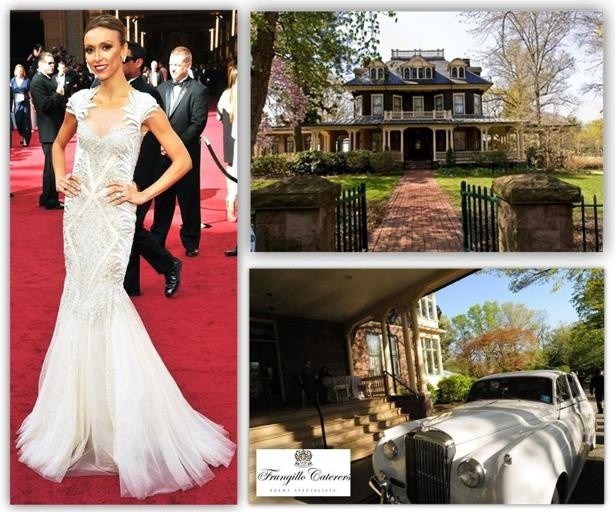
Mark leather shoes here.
[20,142,27,146]
[188,249,198,256]
[165,258,179,295]
[224,249,237,256]
[40,202,64,209]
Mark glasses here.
[48,62,55,64]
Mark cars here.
[366,366,600,505]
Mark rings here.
[114,192,122,199]
[66,173,72,179]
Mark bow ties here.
[172,81,183,87]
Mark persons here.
[11,13,237,500]
[297,359,322,410]
[318,366,333,405]
[262,366,280,409]
[250,361,267,411]
[590,366,604,413]
[10,42,237,256]
[122,40,183,297]
